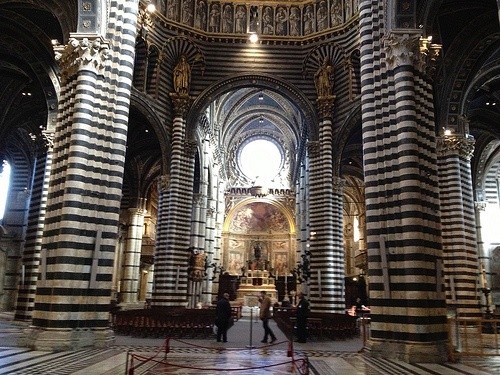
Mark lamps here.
[143,3,156,17]
[248,33,259,43]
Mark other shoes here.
[218,339,229,342]
[295,338,307,343]
[261,336,278,345]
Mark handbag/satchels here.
[212,324,219,336]
[225,316,234,330]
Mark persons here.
[294,292,310,344]
[256,290,278,343]
[217,293,232,342]
